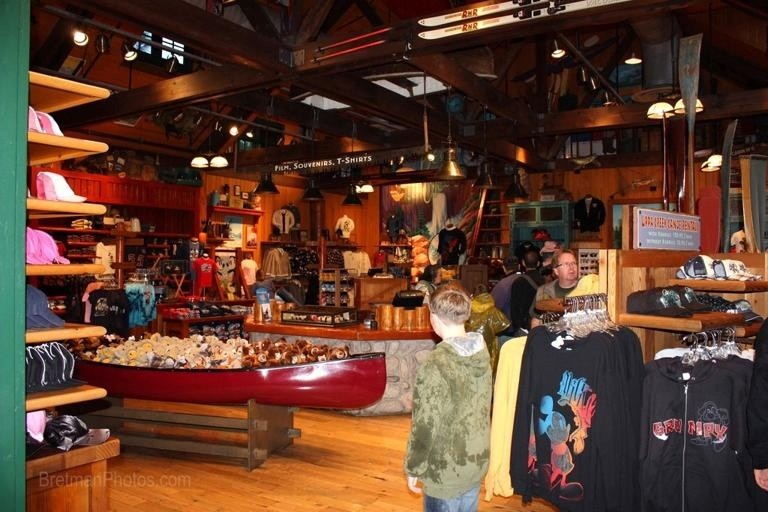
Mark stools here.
[170,272,187,299]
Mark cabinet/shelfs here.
[31,167,206,337]
[507,201,570,254]
[26,385,108,411]
[156,299,257,343]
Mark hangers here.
[664,326,747,363]
[544,294,624,341]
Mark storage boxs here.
[160,260,189,275]
[356,277,408,310]
[25,437,120,512]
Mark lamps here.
[343,182,361,205]
[710,150,724,168]
[503,118,529,198]
[69,17,89,45]
[549,22,601,27]
[255,129,279,193]
[228,120,239,136]
[648,94,674,210]
[122,43,138,61]
[589,73,598,89]
[304,177,323,198]
[435,84,466,181]
[246,125,255,138]
[472,105,499,191]
[190,125,228,167]
[602,90,613,107]
[625,47,640,65]
[355,177,374,193]
[551,37,566,58]
[581,64,590,82]
[418,75,436,163]
[700,157,720,171]
[677,94,703,212]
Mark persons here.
[468,283,509,383]
[529,248,581,328]
[241,252,257,297]
[405,283,491,511]
[418,265,442,298]
[252,268,272,318]
[746,314,768,511]
[514,251,547,336]
[491,256,522,346]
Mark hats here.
[25,433,42,462]
[44,415,111,451]
[626,287,690,320]
[36,172,88,203]
[36,110,64,139]
[25,283,66,330]
[677,255,719,280]
[28,106,42,134]
[668,284,707,314]
[716,258,756,282]
[26,411,44,439]
[32,228,71,265]
[716,300,765,325]
[26,226,53,266]
[25,341,89,395]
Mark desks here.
[242,321,443,415]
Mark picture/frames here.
[234,185,241,196]
[378,180,453,246]
[213,250,235,293]
[242,191,249,199]
[246,226,258,249]
[215,215,247,252]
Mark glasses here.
[553,262,581,269]
